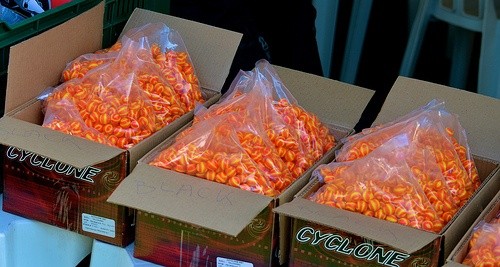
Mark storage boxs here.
[276,74,500,267]
[0,0,244,247]
[444,192,500,267]
[0,1,172,117]
[107,62,376,267]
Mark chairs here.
[400,0,500,96]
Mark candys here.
[40,38,205,149]
[305,121,483,233]
[147,88,335,197]
[461,218,500,267]
